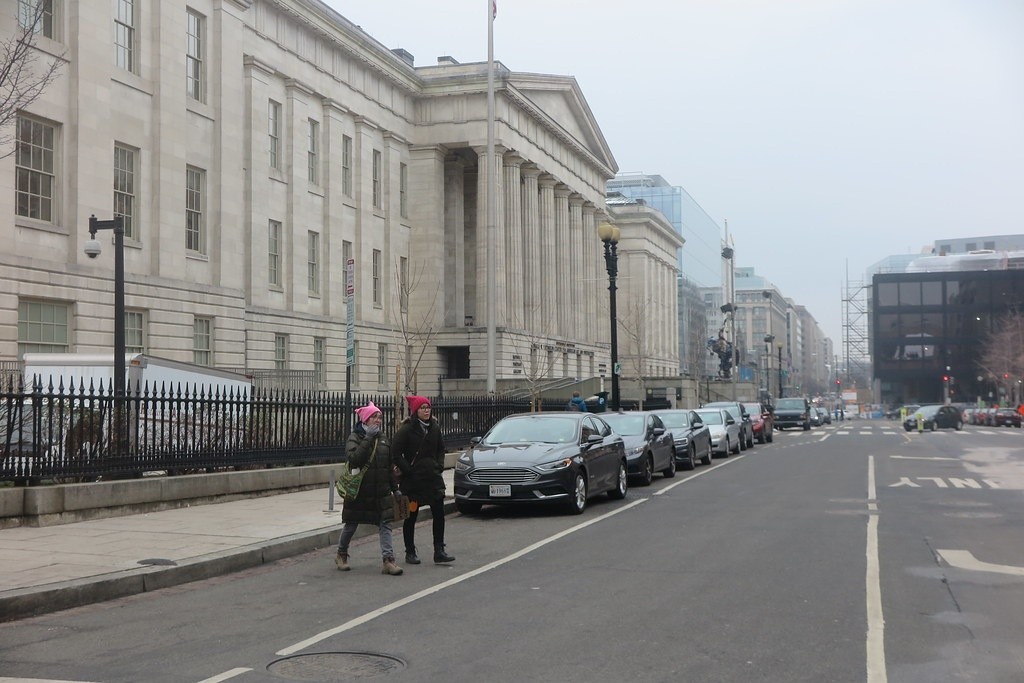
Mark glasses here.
[418,406,432,411]
[369,414,382,421]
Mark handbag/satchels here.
[392,495,410,520]
[336,461,366,502]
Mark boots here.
[403,520,421,564]
[432,518,455,562]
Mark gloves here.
[394,490,403,502]
[365,425,379,437]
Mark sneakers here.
[335,551,350,571]
[383,555,404,575]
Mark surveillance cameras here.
[84,241,102,258]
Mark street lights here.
[777,342,783,398]
[598,216,622,411]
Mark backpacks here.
[571,399,583,411]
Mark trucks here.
[0,352,253,474]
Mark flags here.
[493,0,497,21]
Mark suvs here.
[774,397,811,431]
[700,401,755,451]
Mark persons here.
[389,395,456,564]
[335,401,403,576]
[565,391,588,412]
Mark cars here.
[991,408,1022,427]
[741,403,774,444]
[903,405,964,432]
[647,409,713,470]
[595,411,676,487]
[453,412,627,516]
[809,407,831,426]
[830,409,854,420]
[962,408,995,425]
[886,408,900,420]
[693,410,741,458]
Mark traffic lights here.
[837,380,840,391]
[943,376,948,387]
[946,360,951,371]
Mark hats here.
[355,401,382,423]
[406,395,432,416]
